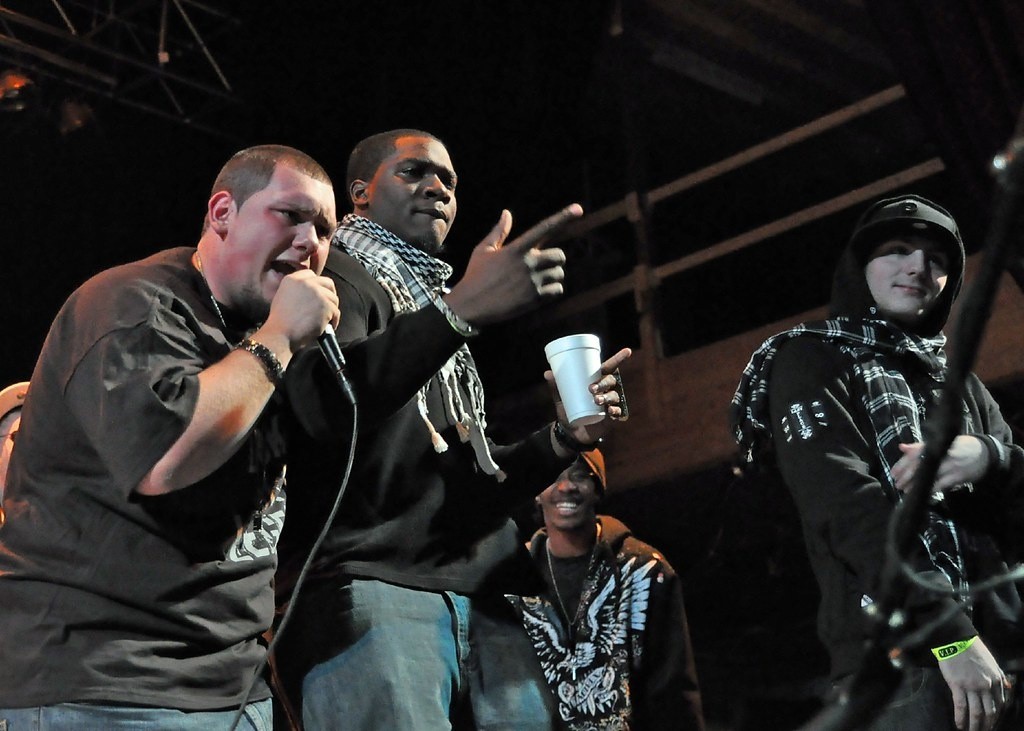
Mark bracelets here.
[551,420,595,458]
[433,295,478,337]
[237,339,286,387]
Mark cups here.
[545,334,607,425]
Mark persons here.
[503,448,705,731]
[546,538,580,626]
[931,636,976,661]
[0,144,340,731]
[729,195,1024,731]
[272,130,582,731]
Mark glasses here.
[0,431,18,442]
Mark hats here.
[580,448,607,490]
[845,194,966,272]
[0,381,31,420]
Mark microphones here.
[310,320,344,374]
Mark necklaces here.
[195,250,226,328]
